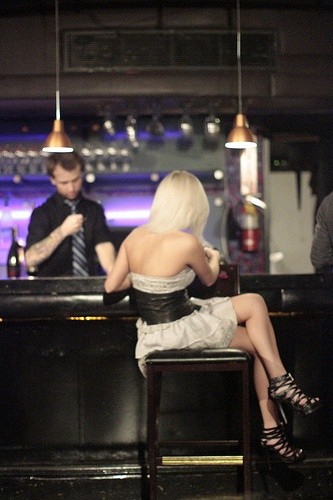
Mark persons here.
[311,192,333,274]
[105,169,323,463]
[23,150,116,278]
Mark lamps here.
[40,1,74,154]
[206,102,223,136]
[224,0,259,150]
[179,101,193,134]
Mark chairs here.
[145,262,256,500]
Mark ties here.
[65,198,90,278]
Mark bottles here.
[6,226,24,281]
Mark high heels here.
[267,372,322,424]
[260,425,306,472]
